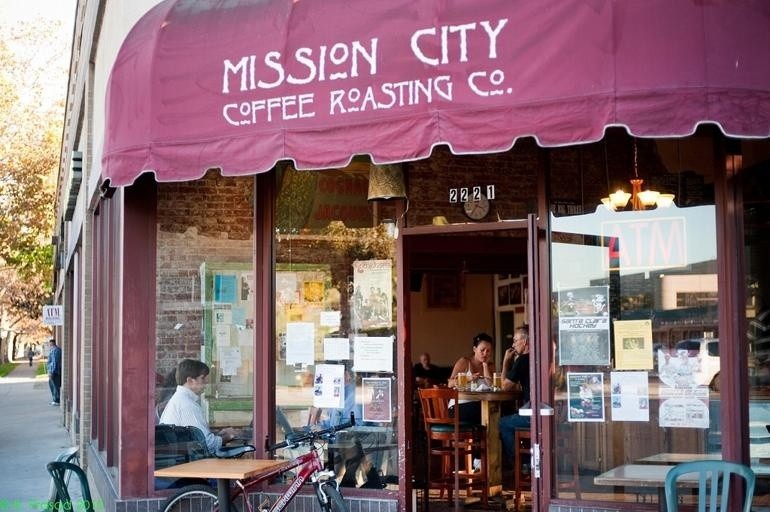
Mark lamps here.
[597,133,678,214]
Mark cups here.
[454,373,467,389]
[492,373,502,388]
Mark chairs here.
[664,461,755,511]
[46,445,94,512]
[417,385,489,510]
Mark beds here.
[457,390,522,500]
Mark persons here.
[47,339,62,407]
[313,350,384,489]
[448,333,494,425]
[500,325,530,474]
[28,346,35,366]
[580,382,592,413]
[414,354,447,383]
[158,358,239,489]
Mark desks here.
[591,461,723,510]
[152,457,290,511]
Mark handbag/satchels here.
[155,423,213,470]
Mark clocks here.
[463,191,491,221]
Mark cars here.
[554,370,770,477]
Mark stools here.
[514,423,581,509]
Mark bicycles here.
[157,410,356,512]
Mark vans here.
[648,338,721,392]
